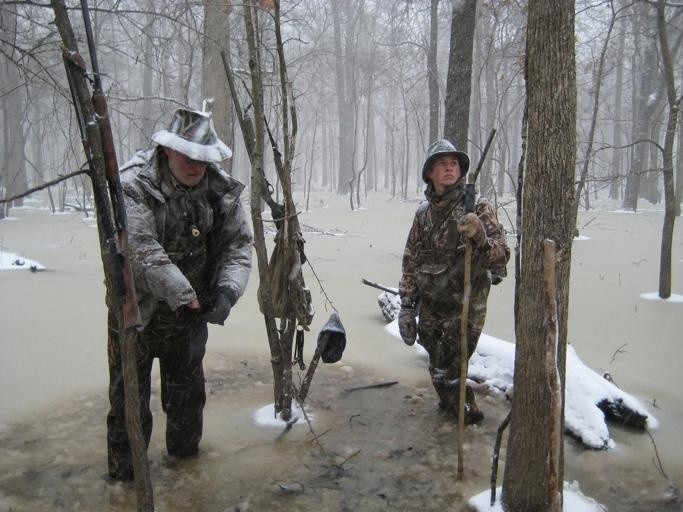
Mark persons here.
[398,139,510,427]
[104,108,254,485]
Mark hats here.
[152,108,232,162]
[422,140,469,183]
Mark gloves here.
[457,213,487,248]
[201,291,231,324]
[399,308,416,345]
